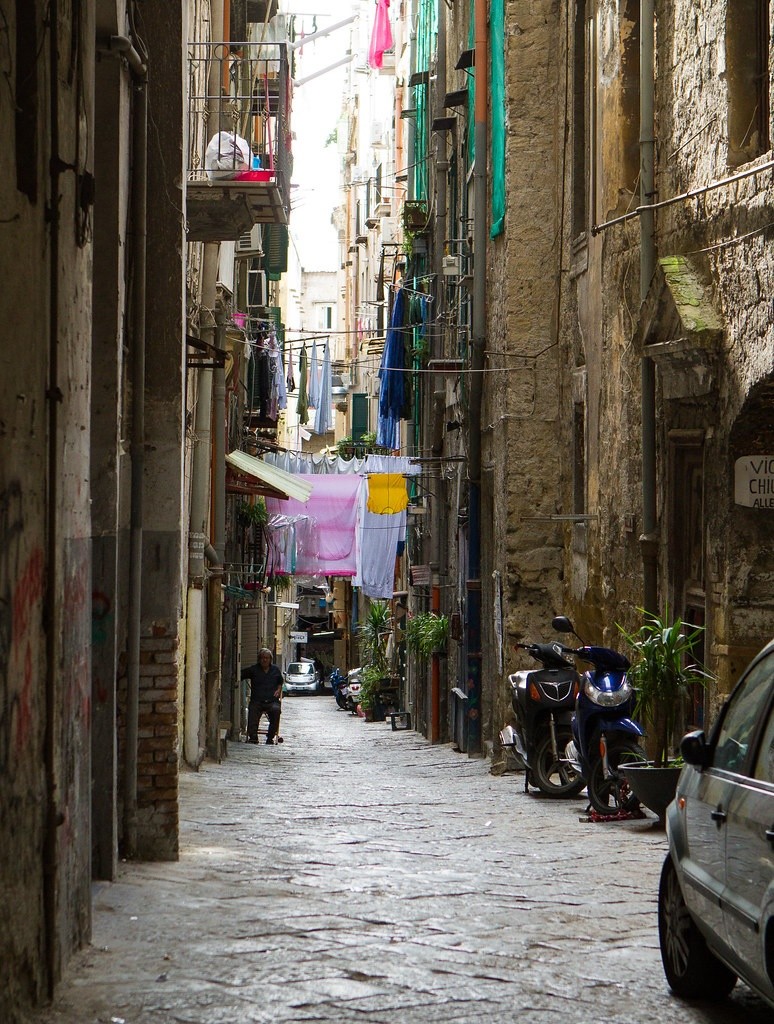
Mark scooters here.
[329,666,361,715]
[551,615,649,819]
[498,640,589,799]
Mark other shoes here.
[248,738,259,744]
[266,739,274,745]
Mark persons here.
[241,648,283,743]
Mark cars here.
[659,638,773,1010]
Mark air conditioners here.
[236,223,263,252]
[246,269,267,308]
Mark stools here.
[390,712,411,731]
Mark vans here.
[283,662,319,697]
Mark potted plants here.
[613,603,720,825]
[355,598,396,722]
[337,432,378,462]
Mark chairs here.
[245,679,282,746]
